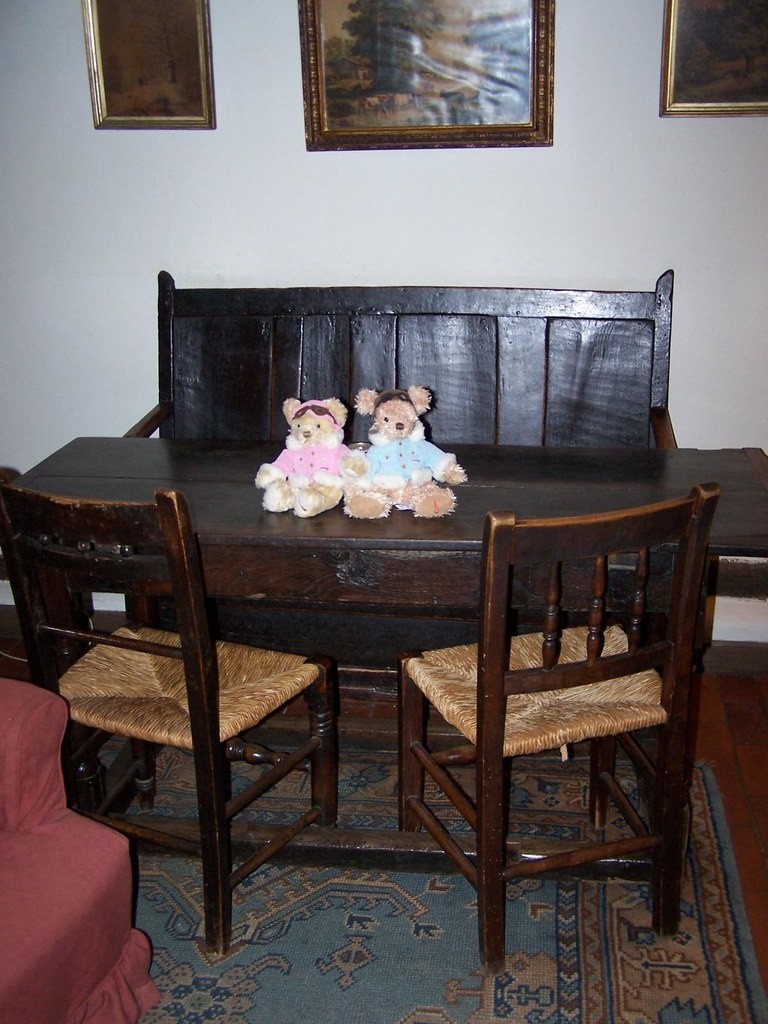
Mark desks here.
[0,436,767,880]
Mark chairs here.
[397,483,720,971]
[0,483,339,956]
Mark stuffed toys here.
[343,385,467,518]
[253,398,358,517]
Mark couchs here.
[0,678,163,1024]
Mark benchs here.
[119,269,707,702]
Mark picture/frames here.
[659,0,768,118]
[81,0,218,131]
[296,1,554,152]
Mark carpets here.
[94,732,768,1024]
